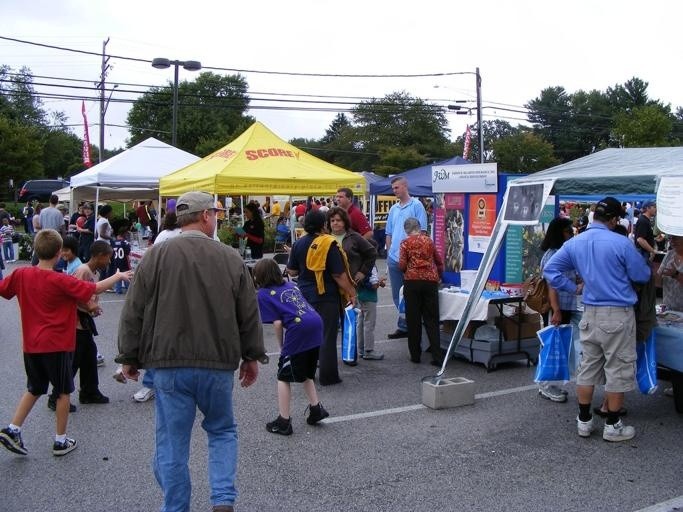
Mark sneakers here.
[53,437,77,456]
[577,414,593,436]
[133,387,155,402]
[593,406,607,418]
[266,415,293,435]
[542,387,565,402]
[604,420,636,441]
[388,329,408,338]
[304,401,329,425]
[1,429,28,455]
[6,261,11,264]
[11,259,16,262]
[431,359,446,368]
[363,352,384,360]
[48,393,76,412]
[539,386,568,396]
[608,407,627,416]
[77,388,109,404]
[410,358,421,363]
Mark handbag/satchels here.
[524,275,551,314]
[535,324,572,386]
[342,305,362,369]
[635,329,658,396]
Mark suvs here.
[18,180,70,206]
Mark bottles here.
[245,246,252,261]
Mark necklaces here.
[674,253,683,265]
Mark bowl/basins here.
[499,283,530,297]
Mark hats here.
[57,204,65,209]
[596,197,626,219]
[176,192,228,216]
[83,202,93,209]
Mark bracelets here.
[670,270,679,278]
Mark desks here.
[655,309,683,410]
[440,289,531,373]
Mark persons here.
[399,218,444,368]
[48,242,113,412]
[654,236,683,311]
[314,200,321,208]
[216,200,224,228]
[616,209,632,233]
[75,202,95,261]
[270,199,280,221]
[28,204,44,232]
[112,231,131,294]
[588,204,600,225]
[327,206,375,289]
[0,216,17,262]
[288,203,297,221]
[627,201,636,218]
[283,210,356,386]
[539,217,576,402]
[153,212,184,243]
[384,178,428,340]
[632,210,640,228]
[24,201,34,234]
[319,201,329,210]
[38,195,65,230]
[320,198,324,202]
[358,240,386,360]
[326,199,330,206]
[56,237,82,275]
[275,217,291,242]
[335,189,373,240]
[69,204,84,233]
[0,202,18,225]
[251,258,329,436]
[0,230,133,456]
[96,204,114,243]
[261,199,270,213]
[144,209,158,239]
[295,201,306,217]
[579,207,589,232]
[543,196,651,443]
[232,204,265,258]
[635,201,665,259]
[113,192,270,511]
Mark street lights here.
[152,57,202,147]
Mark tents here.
[155,122,367,251]
[68,138,203,242]
[435,145,683,385]
[355,170,387,228]
[370,155,470,287]
